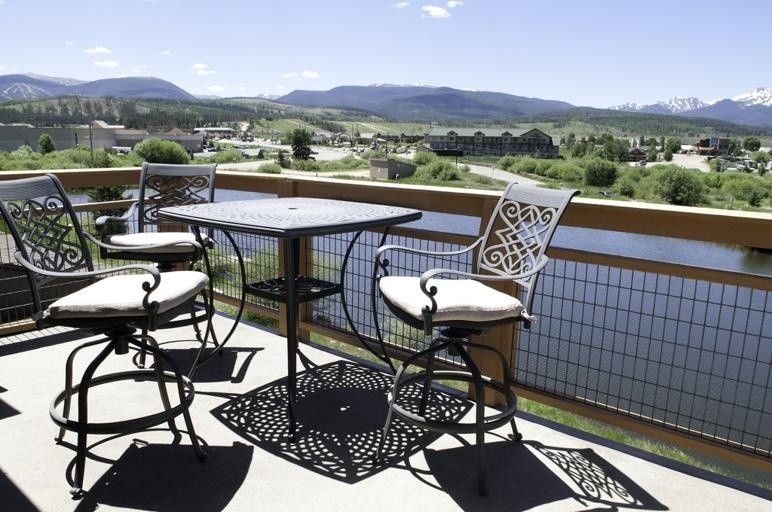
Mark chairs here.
[369,180,580,497]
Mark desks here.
[155,192,423,442]
